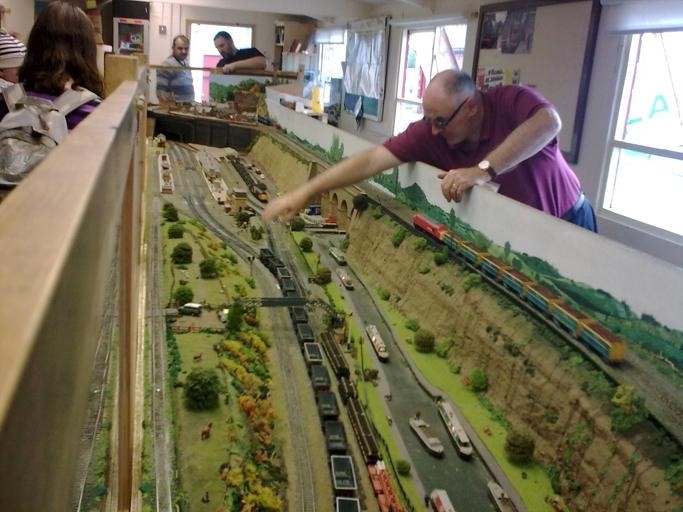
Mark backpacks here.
[0,83,102,185]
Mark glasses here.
[422,97,469,129]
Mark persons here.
[260,69,597,234]
[15,0,105,134]
[213,31,267,74]
[155,35,194,103]
[0,30,28,84]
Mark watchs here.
[478,160,497,180]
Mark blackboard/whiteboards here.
[343,15,393,122]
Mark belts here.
[560,187,585,221]
